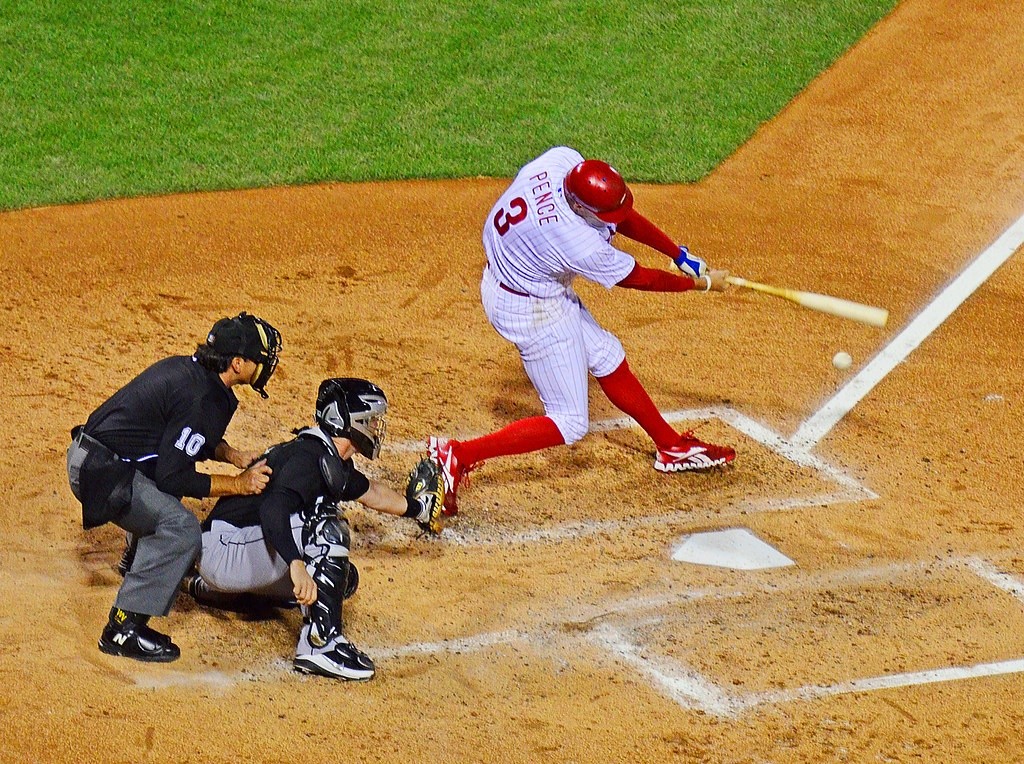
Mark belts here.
[75,432,94,453]
[200,519,212,534]
[486,260,529,298]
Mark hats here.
[205,317,266,356]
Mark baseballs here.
[833,352,852,369]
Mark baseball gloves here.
[402,455,445,539]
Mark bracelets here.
[701,275,711,293]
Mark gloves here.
[669,245,707,279]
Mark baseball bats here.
[723,277,888,326]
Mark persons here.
[198,378,445,682]
[408,146,736,517]
[67,312,282,662]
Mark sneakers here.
[118,548,134,575]
[180,570,210,601]
[97,607,180,662]
[294,623,375,681]
[654,421,735,472]
[426,435,485,517]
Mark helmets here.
[316,378,388,460]
[568,160,634,222]
